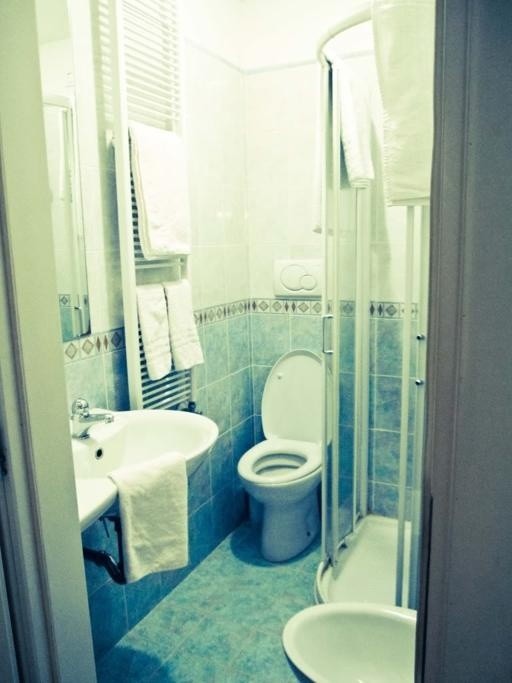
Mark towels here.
[135,283,172,380]
[312,51,375,237]
[112,120,193,261]
[369,1,435,207]
[107,452,189,584]
[162,279,205,371]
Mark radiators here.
[108,0,197,411]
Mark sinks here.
[69,408,219,533]
[282,603,417,683]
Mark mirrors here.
[42,102,91,342]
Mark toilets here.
[236,351,341,562]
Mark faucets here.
[71,398,114,439]
